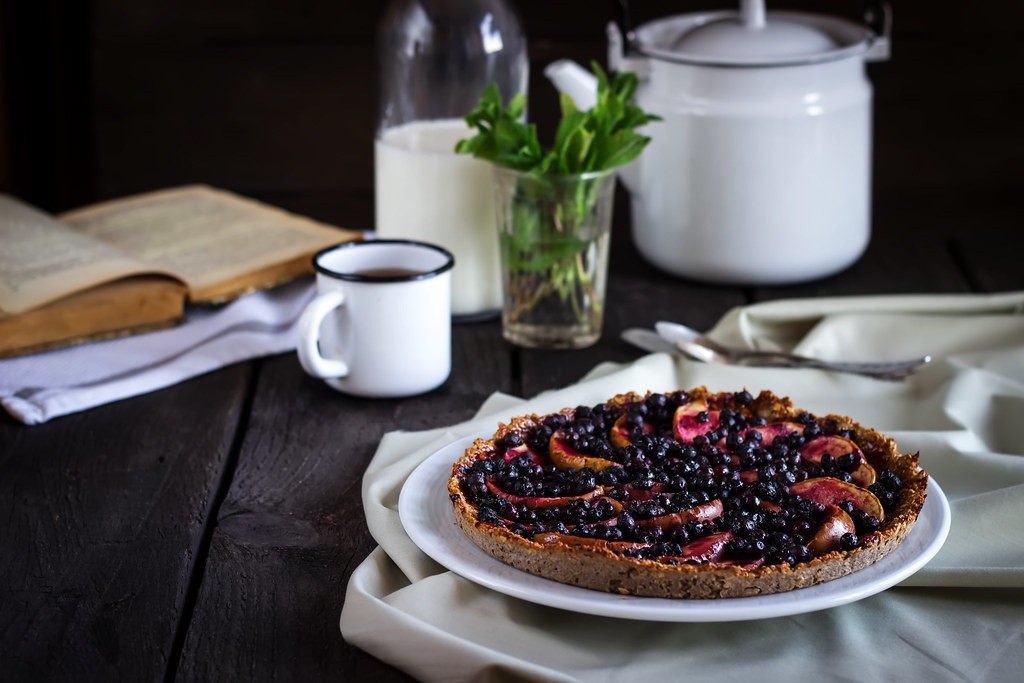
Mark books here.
[1,184,364,359]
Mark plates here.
[396,430,951,624]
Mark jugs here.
[542,0,891,285]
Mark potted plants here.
[453,61,664,353]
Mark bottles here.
[373,0,531,326]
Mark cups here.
[297,238,454,399]
[484,162,616,350]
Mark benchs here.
[94,0,1024,243]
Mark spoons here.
[657,320,933,374]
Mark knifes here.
[621,327,920,380]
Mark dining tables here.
[0,230,1024,683]
